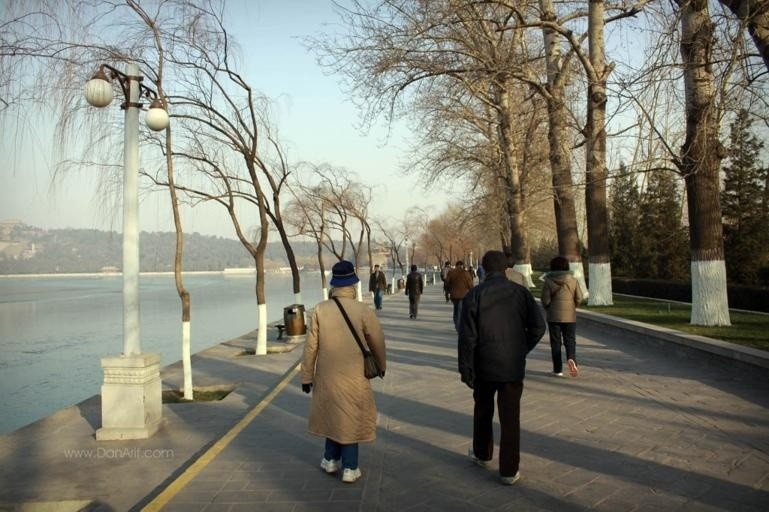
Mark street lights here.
[84,55,171,442]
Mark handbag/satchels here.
[363,350,377,379]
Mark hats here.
[482,250,508,271]
[330,261,359,287]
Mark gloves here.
[302,383,313,394]
[379,371,385,379]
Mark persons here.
[457,249,546,486]
[300,260,387,484]
[501,253,532,294]
[368,263,386,312]
[467,265,476,283]
[444,261,474,331]
[440,259,454,304]
[539,255,584,378]
[476,264,488,285]
[405,264,424,321]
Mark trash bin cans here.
[397,279,404,288]
[284,304,306,336]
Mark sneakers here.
[470,453,492,468]
[568,359,578,377]
[321,458,338,472]
[342,466,362,482]
[551,371,563,376]
[502,471,520,485]
